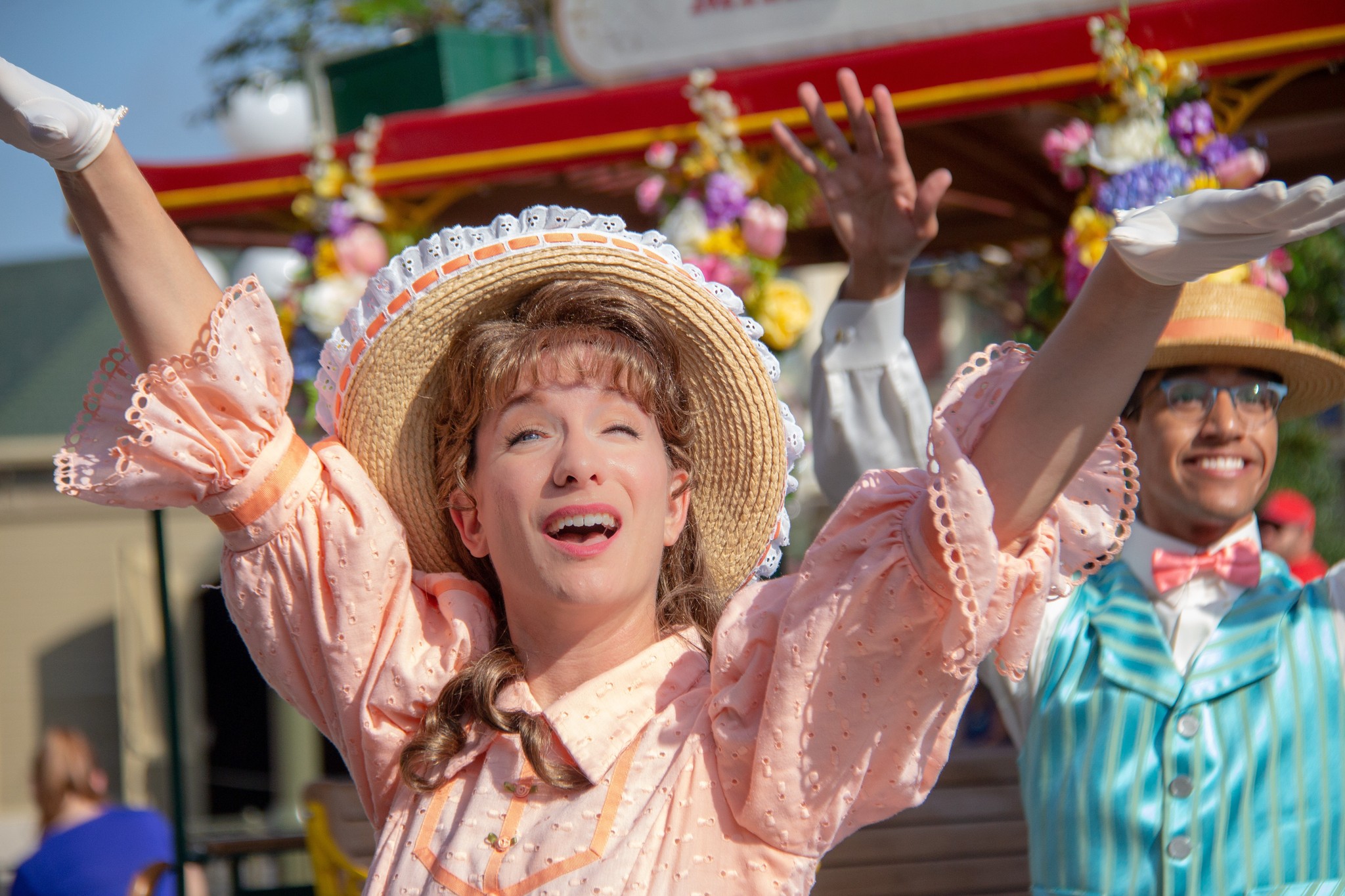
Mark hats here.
[1146,281,1345,424]
[1258,490,1316,532]
[314,205,805,601]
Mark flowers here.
[634,61,815,351]
[234,110,421,387]
[952,11,1345,372]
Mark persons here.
[7,729,210,896]
[0,59,1345,896]
[1256,488,1328,587]
[767,67,1345,896]
[950,684,1019,753]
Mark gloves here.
[1104,177,1345,285]
[0,57,128,174]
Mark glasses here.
[1126,381,1289,424]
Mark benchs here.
[304,754,1032,896]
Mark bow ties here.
[1152,538,1261,592]
